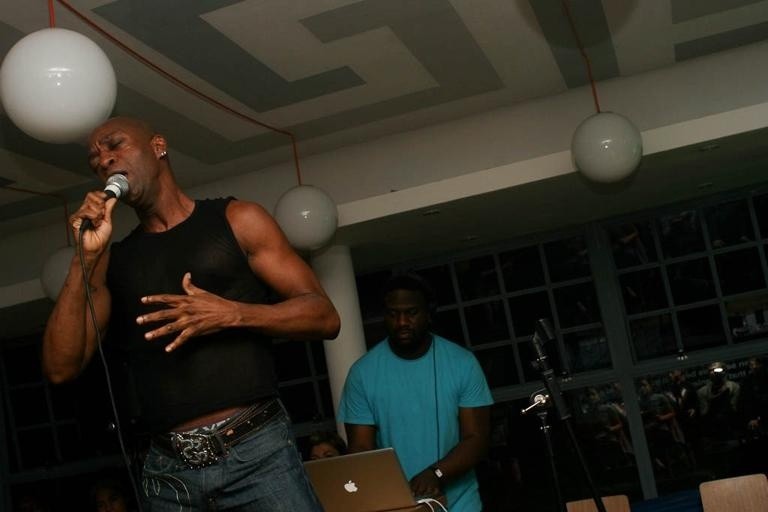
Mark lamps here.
[0,0,118,146]
[274,135,338,252]
[42,197,78,302]
[571,57,643,185]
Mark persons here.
[336,274,495,512]
[298,430,347,464]
[564,354,768,489]
[93,481,130,512]
[40,116,341,512]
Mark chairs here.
[697,472,768,512]
[566,494,631,512]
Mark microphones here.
[76,170,130,237]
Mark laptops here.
[297,449,423,510]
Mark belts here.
[171,402,282,469]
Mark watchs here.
[429,465,445,483]
